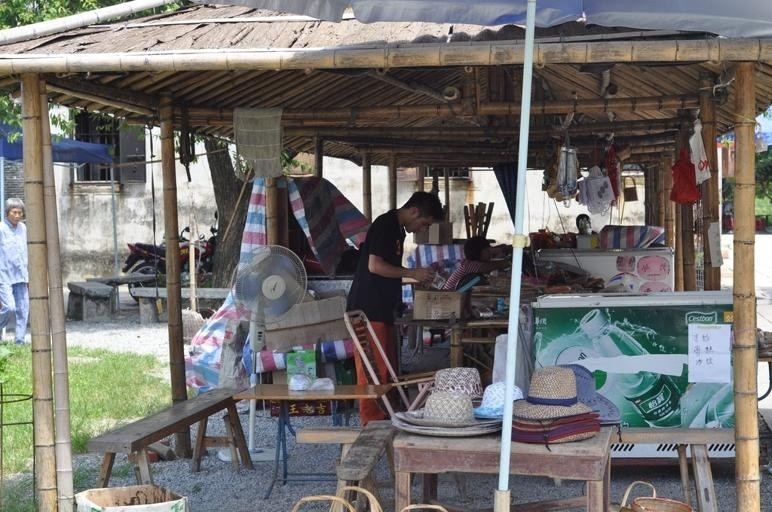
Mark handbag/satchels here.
[578,166,617,216]
[669,146,701,205]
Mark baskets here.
[618,480,694,511]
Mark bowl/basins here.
[288,375,336,391]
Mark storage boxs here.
[412,281,470,322]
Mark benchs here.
[67,274,230,324]
[603,428,736,512]
[297,419,401,512]
[85,387,255,491]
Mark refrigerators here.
[532,292,758,477]
[532,247,675,293]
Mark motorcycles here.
[122,209,218,305]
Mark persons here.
[443,236,512,291]
[0,196,29,344]
[346,190,443,428]
[576,214,591,236]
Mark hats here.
[511,362,622,445]
[391,366,525,438]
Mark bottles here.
[496,296,505,312]
[422,326,434,347]
[581,308,685,428]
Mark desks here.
[231,383,392,503]
[391,425,612,512]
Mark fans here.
[216,245,308,462]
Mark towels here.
[233,106,285,183]
